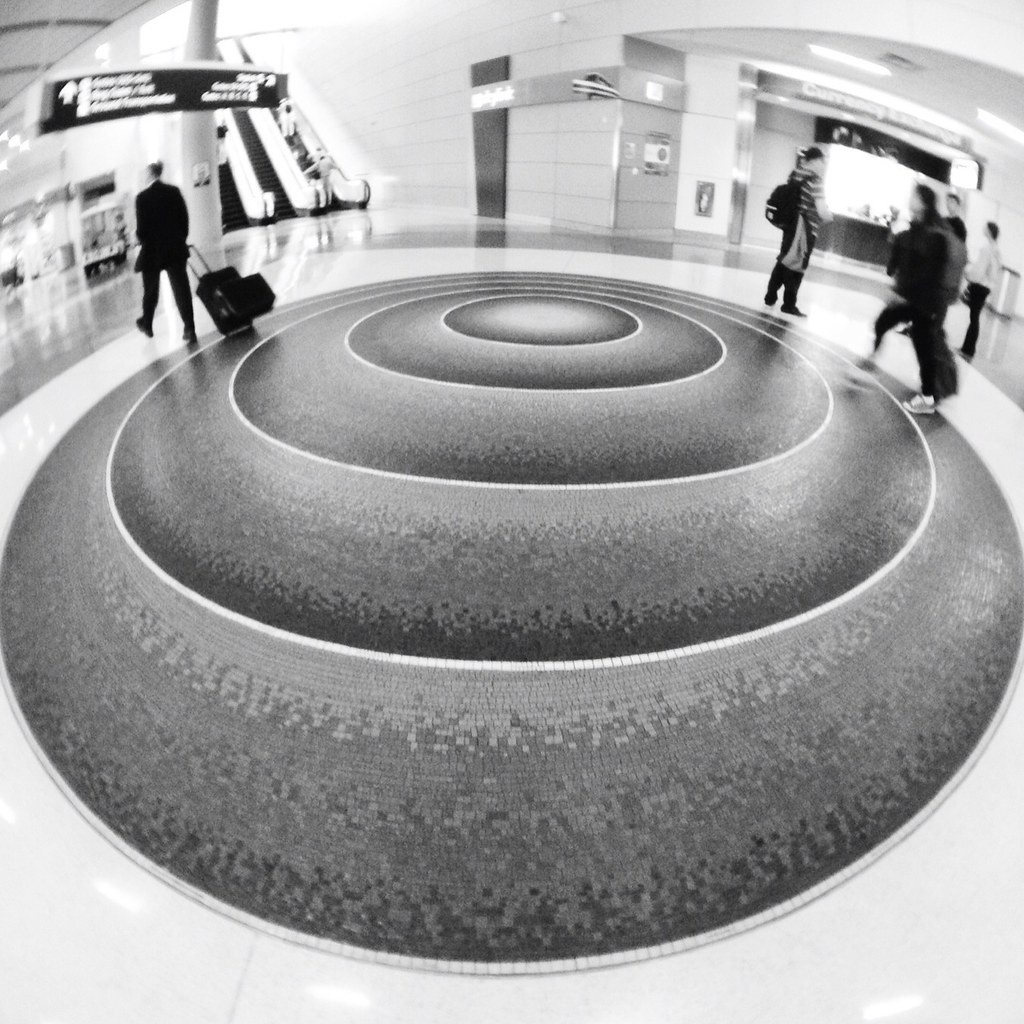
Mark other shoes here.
[765,297,776,307]
[955,349,972,362]
[184,328,198,342]
[781,305,807,317]
[137,316,153,337]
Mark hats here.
[802,148,822,160]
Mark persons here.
[277,97,336,207]
[135,161,197,343]
[856,183,1000,414]
[765,148,834,317]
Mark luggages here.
[186,245,275,335]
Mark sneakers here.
[904,392,936,414]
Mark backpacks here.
[766,173,817,229]
[928,223,966,302]
